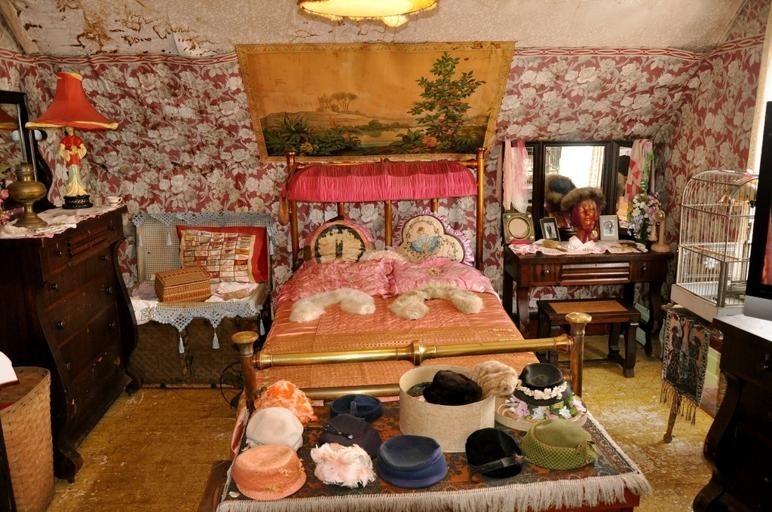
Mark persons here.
[545,175,578,230]
[59,125,90,197]
[546,224,553,237]
[560,187,607,243]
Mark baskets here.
[154,265,213,305]
[0,365,57,512]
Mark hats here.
[317,412,383,461]
[243,407,305,452]
[308,442,378,490]
[519,417,603,471]
[422,368,482,407]
[230,443,308,503]
[253,380,321,425]
[464,428,529,480]
[374,434,450,490]
[559,186,609,210]
[462,360,519,401]
[329,393,383,422]
[513,363,573,407]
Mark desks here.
[501,240,673,359]
[657,302,728,444]
[211,391,650,512]
[691,311,772,512]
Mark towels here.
[471,360,518,398]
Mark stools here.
[535,297,642,379]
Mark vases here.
[638,218,649,240]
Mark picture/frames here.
[232,40,517,168]
[599,214,619,241]
[539,217,560,241]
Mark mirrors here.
[533,139,617,235]
[1,89,41,212]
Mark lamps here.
[295,0,444,31]
[26,68,123,213]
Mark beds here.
[230,148,595,418]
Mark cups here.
[106,195,122,207]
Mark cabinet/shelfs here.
[0,198,141,484]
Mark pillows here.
[391,208,476,269]
[309,217,376,265]
[173,222,270,285]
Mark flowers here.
[625,190,665,235]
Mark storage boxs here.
[129,210,274,389]
[398,364,495,453]
